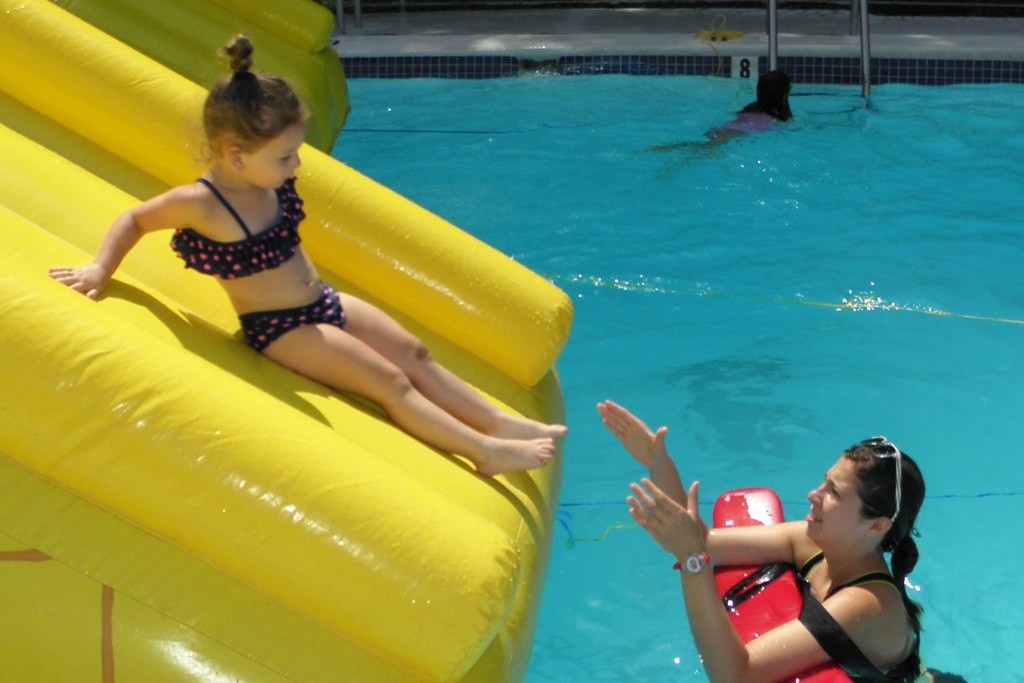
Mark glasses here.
[860,435,903,524]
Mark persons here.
[597,400,925,683]
[741,70,794,123]
[47,35,571,478]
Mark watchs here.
[673,553,709,573]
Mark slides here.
[0,0,574,683]
